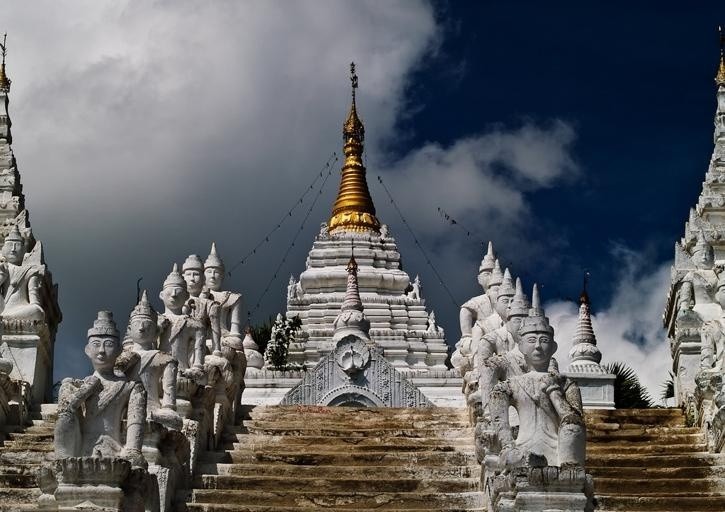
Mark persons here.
[681,247,725,382]
[0,332,15,424]
[287,273,297,299]
[0,224,47,321]
[428,310,436,330]
[450,240,586,467]
[55,241,247,468]
[410,274,421,299]
